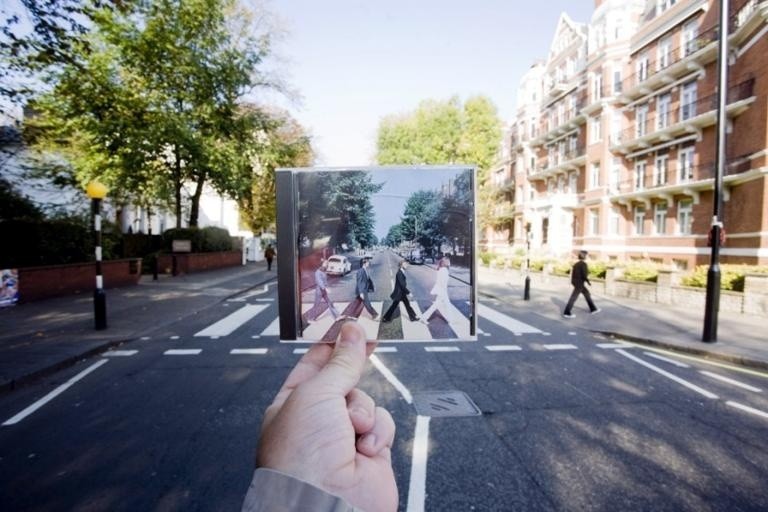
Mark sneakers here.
[348,315,358,321]
[373,316,380,322]
[307,320,317,325]
[563,311,576,318]
[590,308,601,315]
[419,318,430,325]
[336,315,346,323]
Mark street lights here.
[83,180,112,334]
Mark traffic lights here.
[525,222,533,235]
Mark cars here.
[323,254,354,280]
[391,245,427,267]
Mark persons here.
[349,259,380,320]
[560,249,602,320]
[239,321,401,510]
[420,258,463,324]
[383,261,421,322]
[264,243,276,271]
[308,259,345,322]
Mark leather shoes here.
[411,317,419,321]
[382,317,392,324]
